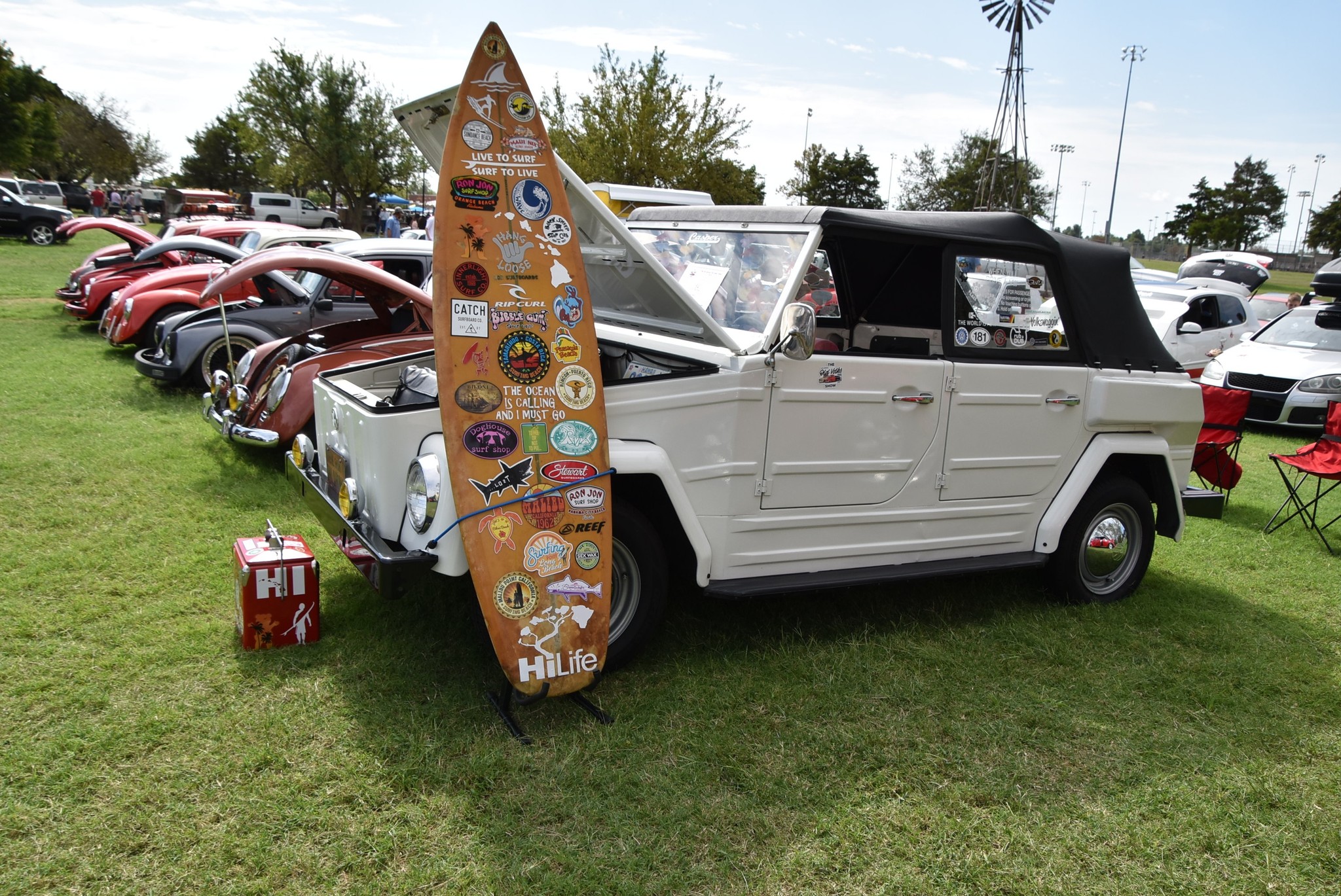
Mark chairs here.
[1262,400,1341,556]
[1189,381,1254,507]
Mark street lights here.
[1076,181,1092,240]
[1140,208,1181,246]
[1266,164,1297,271]
[1094,44,1149,247]
[1297,153,1326,272]
[1049,145,1077,235]
[1289,190,1312,254]
[1091,210,1097,237]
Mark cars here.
[246,192,340,228]
[20,179,68,211]
[1245,292,1331,328]
[86,180,170,217]
[58,183,91,214]
[1032,268,1178,336]
[0,178,31,205]
[165,189,231,218]
[98,224,371,353]
[0,184,80,246]
[198,244,464,467]
[1133,251,1271,385]
[964,272,1043,325]
[64,217,309,322]
[1198,257,1341,433]
[281,205,1204,606]
[54,217,249,304]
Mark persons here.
[125,191,135,215]
[91,186,104,219]
[110,189,123,215]
[134,189,143,212]
[1285,293,1301,310]
[375,205,435,241]
[956,256,982,273]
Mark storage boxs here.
[1180,485,1225,519]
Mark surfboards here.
[424,20,612,704]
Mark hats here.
[395,206,405,215]
